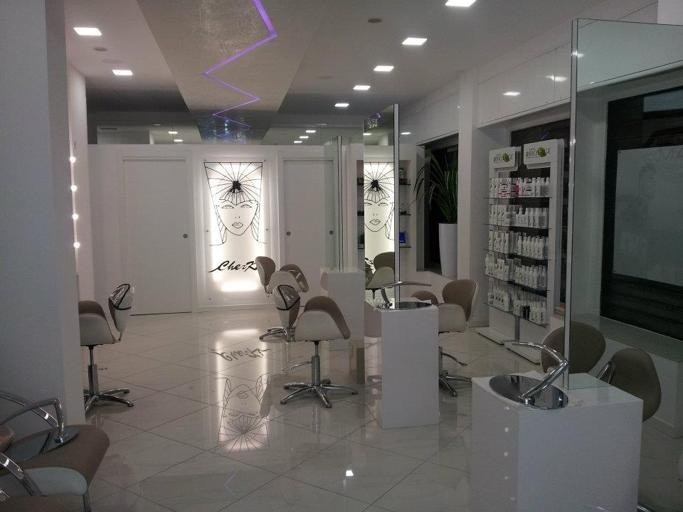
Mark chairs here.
[3,397,109,510]
[78,284,134,412]
[364,252,394,307]
[540,321,661,422]
[255,257,358,408]
[411,280,480,396]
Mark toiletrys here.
[400,168,408,242]
[486,173,550,326]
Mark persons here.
[628,165,656,244]
[218,345,266,450]
[364,173,396,276]
[212,172,266,261]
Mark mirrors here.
[361,105,399,312]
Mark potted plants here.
[412,148,457,278]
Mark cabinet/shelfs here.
[476,138,565,364]
